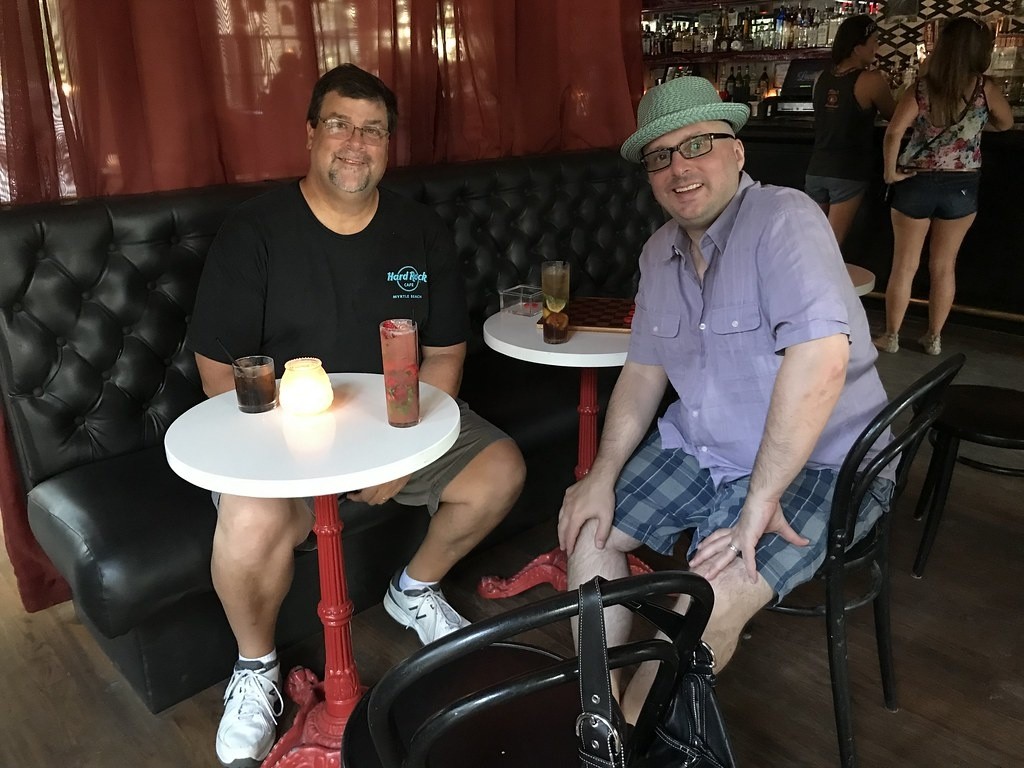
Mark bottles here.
[757,66,770,99]
[742,65,750,96]
[733,66,743,98]
[725,67,735,98]
[638,2,875,56]
[749,72,758,96]
[719,66,728,93]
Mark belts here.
[904,169,977,172]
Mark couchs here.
[0,149,670,715]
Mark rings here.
[727,543,741,557]
[382,497,387,502]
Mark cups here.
[379,317,420,429]
[539,261,571,343]
[278,356,334,413]
[232,356,277,414]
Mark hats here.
[620,75,750,164]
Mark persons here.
[557,76,901,725]
[872,16,1015,356]
[806,14,897,250]
[189,63,527,767]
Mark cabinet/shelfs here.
[635,0,875,121]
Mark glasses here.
[315,114,391,147]
[638,133,736,173]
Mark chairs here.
[891,383,1024,578]
[341,572,735,768]
[640,350,966,768]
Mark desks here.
[480,296,655,600]
[164,372,463,768]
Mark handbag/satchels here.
[575,575,726,768]
[874,174,897,209]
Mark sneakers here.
[215,659,285,768]
[382,567,473,646]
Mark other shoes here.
[874,333,899,354]
[917,331,941,356]
